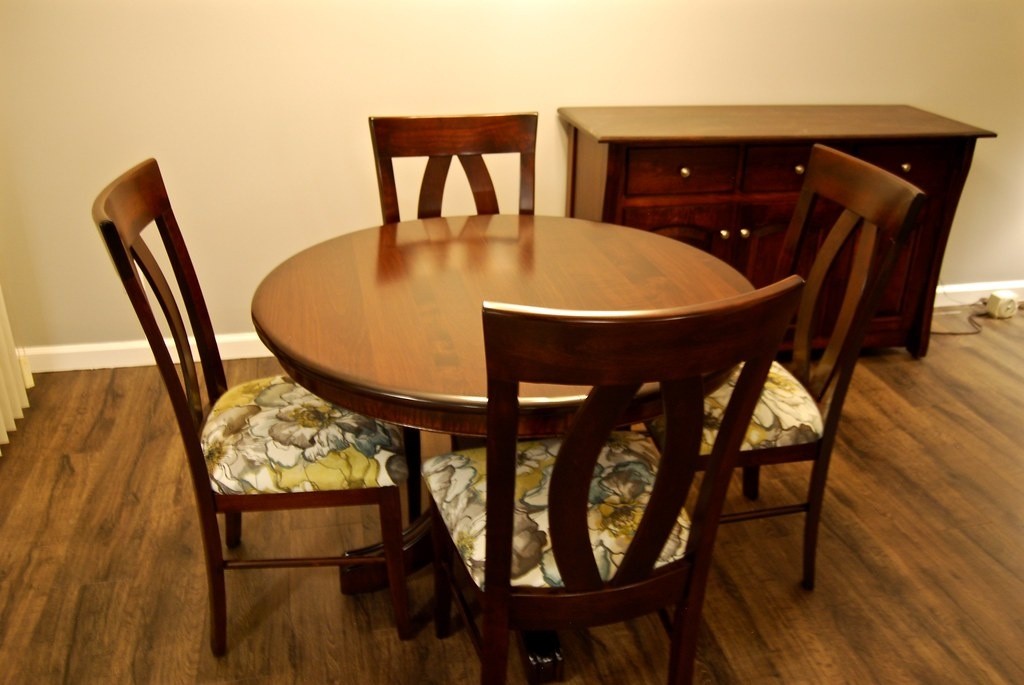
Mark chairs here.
[640,144,925,594]
[367,114,540,522]
[423,274,807,685]
[92,157,416,660]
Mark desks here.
[252,213,754,593]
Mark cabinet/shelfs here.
[556,103,997,360]
